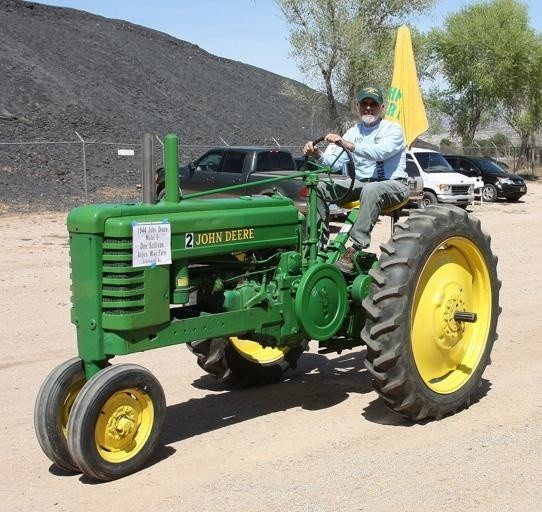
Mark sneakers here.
[333,247,360,275]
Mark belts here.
[360,177,409,183]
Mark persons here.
[301,87,409,274]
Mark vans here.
[405,147,475,210]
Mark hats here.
[358,85,383,106]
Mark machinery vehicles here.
[33,133,503,481]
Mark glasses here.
[359,99,378,108]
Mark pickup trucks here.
[156,145,352,220]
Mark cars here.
[445,154,527,202]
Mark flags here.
[384,24,430,147]
